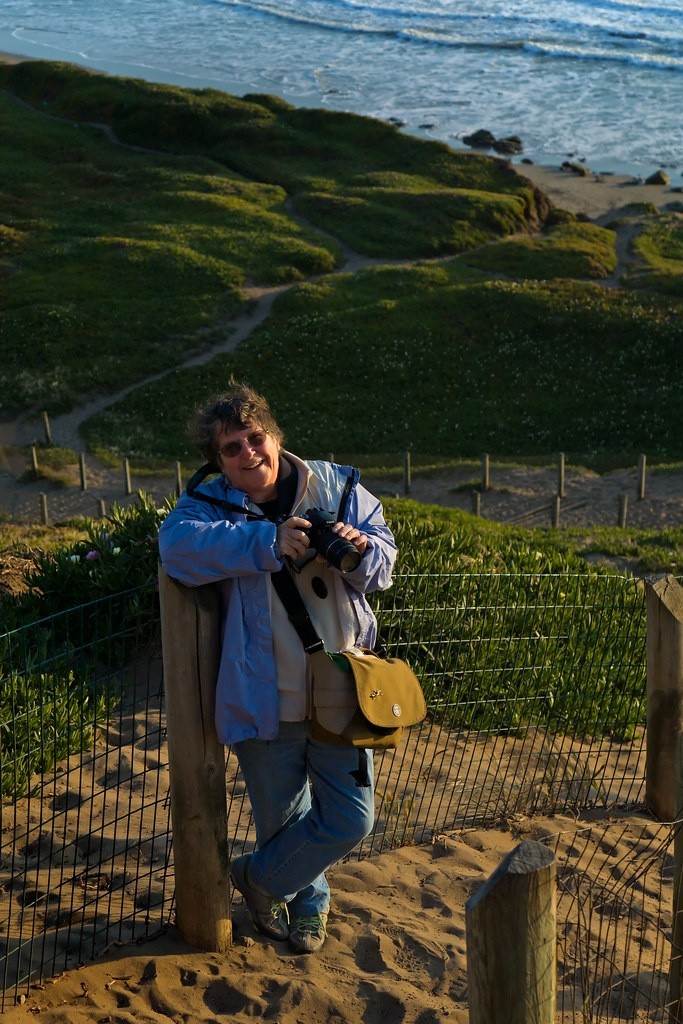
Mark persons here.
[157,376,399,955]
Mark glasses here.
[218,430,270,457]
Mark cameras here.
[283,506,362,574]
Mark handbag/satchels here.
[311,649,427,748]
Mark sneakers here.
[230,853,290,942]
[289,896,330,954]
